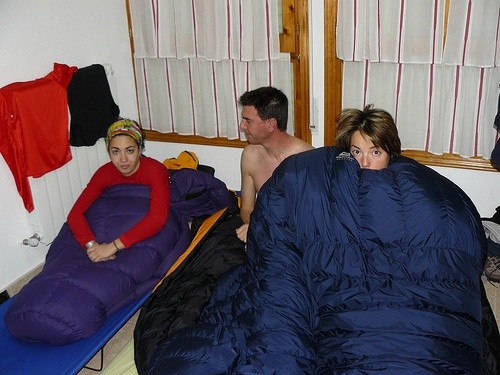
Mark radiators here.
[0,64,122,247]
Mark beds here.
[0,185,236,375]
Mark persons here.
[332,103,401,171]
[67,119,171,263]
[235,86,317,243]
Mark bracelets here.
[86,240,96,248]
[113,240,120,251]
